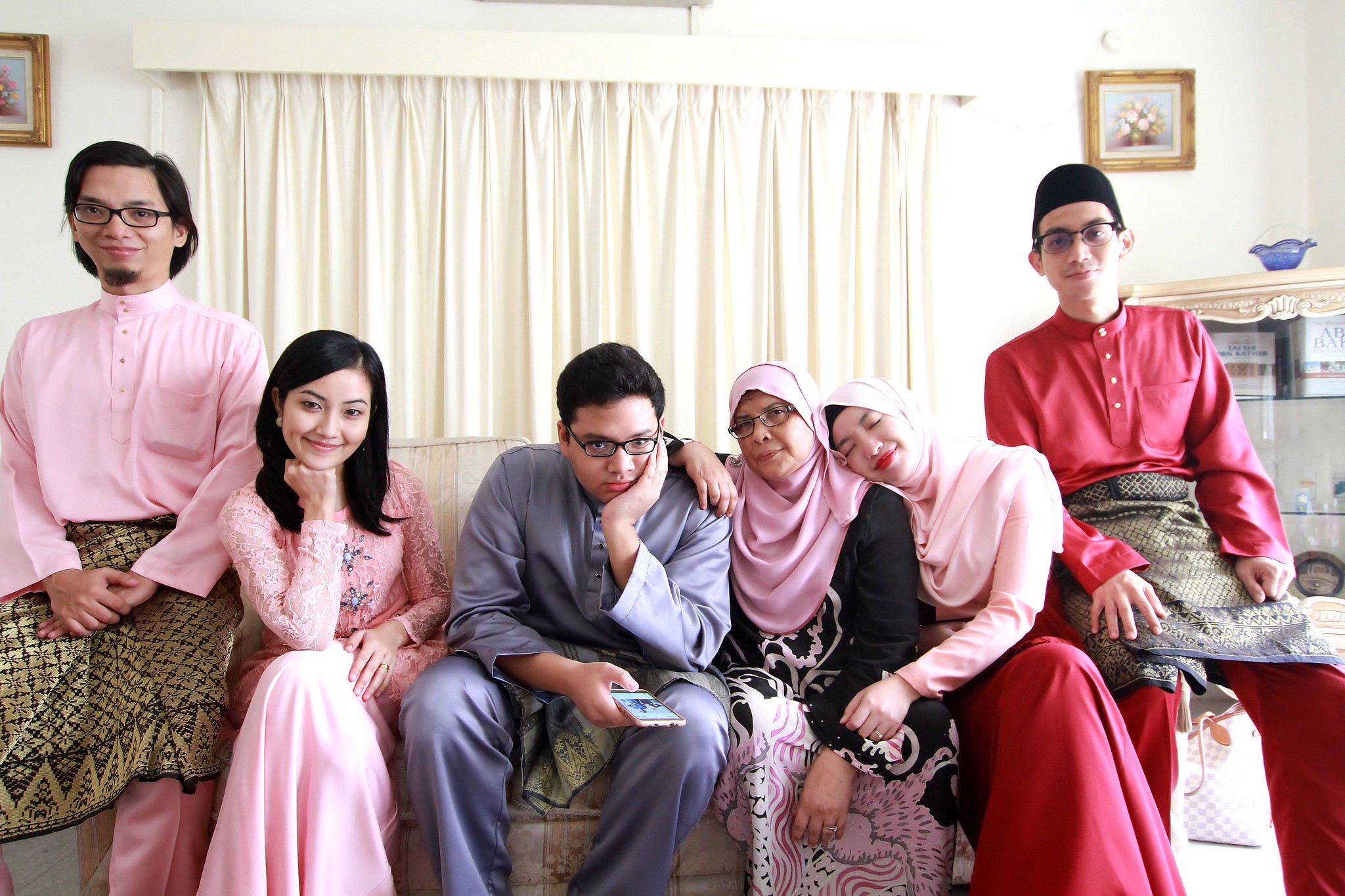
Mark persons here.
[201,326,455,896]
[405,341,740,896]
[808,377,1188,896]
[676,363,959,896]
[0,141,270,896]
[982,165,1345,896]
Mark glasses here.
[728,405,795,439]
[565,420,660,457]
[69,203,170,228]
[1034,221,1124,255]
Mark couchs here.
[0,435,749,896]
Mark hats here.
[1032,164,1121,227]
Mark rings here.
[380,663,390,672]
[822,825,837,831]
[874,728,883,739]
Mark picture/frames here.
[0,33,52,148]
[1087,69,1196,172]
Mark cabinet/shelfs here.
[1118,266,1345,601]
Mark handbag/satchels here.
[1185,701,1271,846]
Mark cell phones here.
[609,687,686,728]
